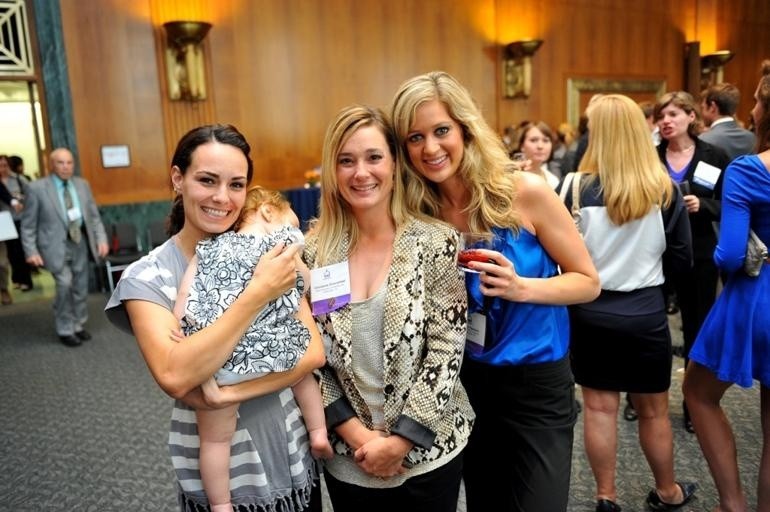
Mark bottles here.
[111,225,120,251]
[135,234,143,252]
[145,223,154,250]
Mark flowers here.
[302,170,321,188]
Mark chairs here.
[146,220,173,252]
[103,223,144,295]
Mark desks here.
[280,186,320,221]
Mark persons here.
[0,155,33,291]
[103,124,323,511]
[20,148,109,347]
[393,72,601,511]
[172,185,335,511]
[506,58,769,511]
[301,105,477,510]
[8,156,31,181]
[0,180,23,305]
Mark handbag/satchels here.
[744,228,768,277]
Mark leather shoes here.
[59,328,92,346]
[683,399,695,433]
[625,401,638,422]
[595,481,696,512]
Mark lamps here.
[701,51,736,96]
[164,20,212,100]
[501,40,543,99]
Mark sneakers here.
[0,282,30,305]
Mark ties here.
[63,178,82,244]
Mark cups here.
[454,229,495,275]
[513,152,528,162]
[676,179,691,197]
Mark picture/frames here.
[566,75,668,128]
